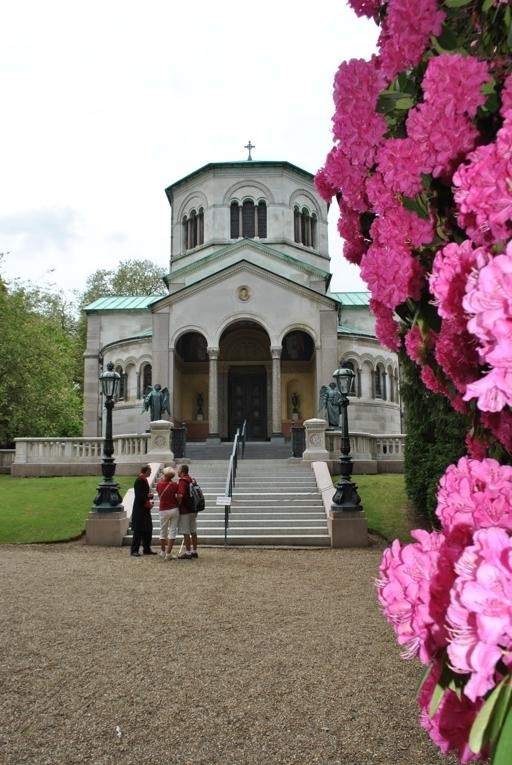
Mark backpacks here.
[179,477,205,513]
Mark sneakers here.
[158,551,166,558]
[177,552,192,559]
[192,552,199,558]
[165,554,177,560]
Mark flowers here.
[312,0,512,765]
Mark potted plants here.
[195,392,205,421]
[290,391,302,421]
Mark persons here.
[176,465,198,560]
[322,382,343,429]
[130,464,158,557]
[156,466,182,560]
[144,383,165,421]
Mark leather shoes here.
[130,551,141,557]
[142,548,158,555]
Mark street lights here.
[92,361,124,511]
[331,360,362,512]
